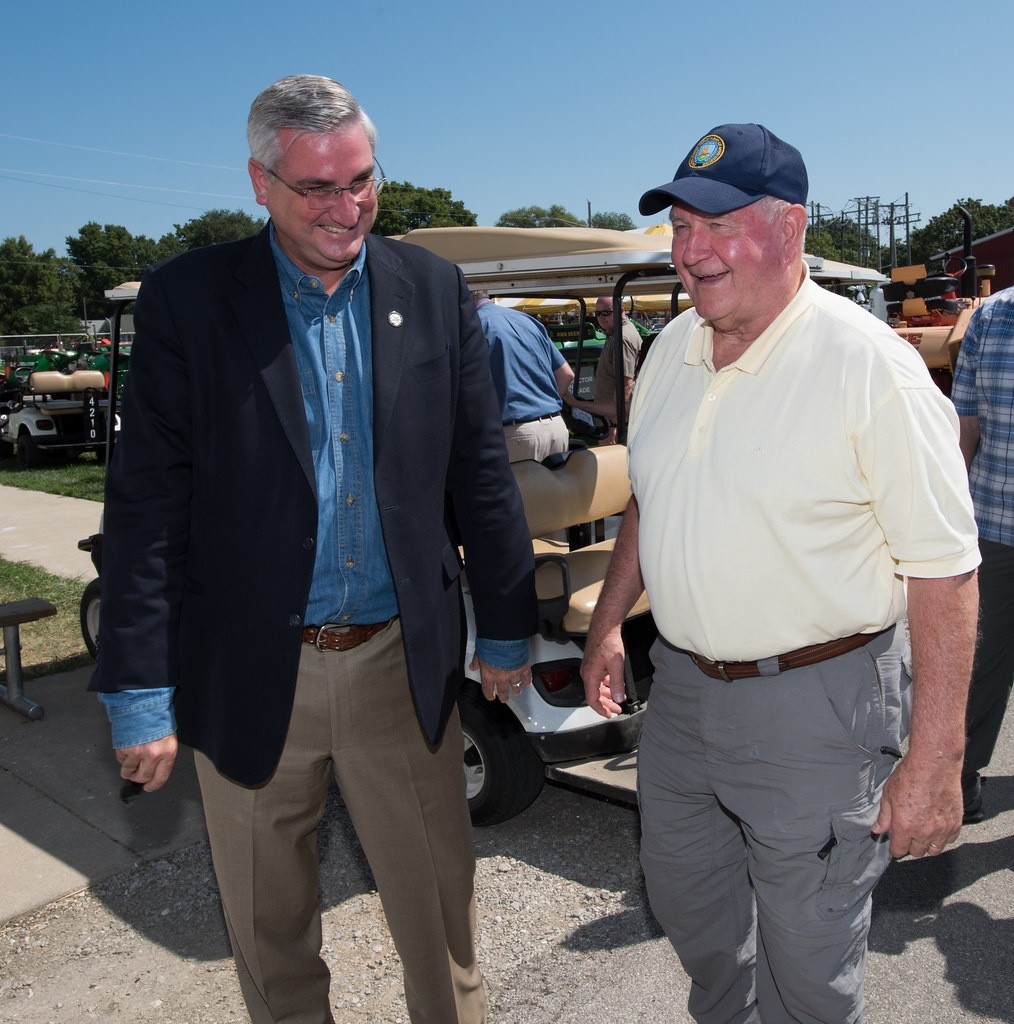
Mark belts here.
[300,620,391,654]
[503,411,561,427]
[690,636,872,682]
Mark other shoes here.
[963,774,984,821]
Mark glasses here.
[263,156,387,209]
[592,309,616,315]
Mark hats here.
[639,123,809,216]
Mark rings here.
[510,679,522,687]
[931,840,945,849]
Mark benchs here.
[509,443,653,641]
[0,597,57,721]
[28,369,105,402]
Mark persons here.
[953,283,1014,825]
[565,296,644,434]
[581,123,982,1024]
[467,285,576,465]
[89,71,536,1022]
